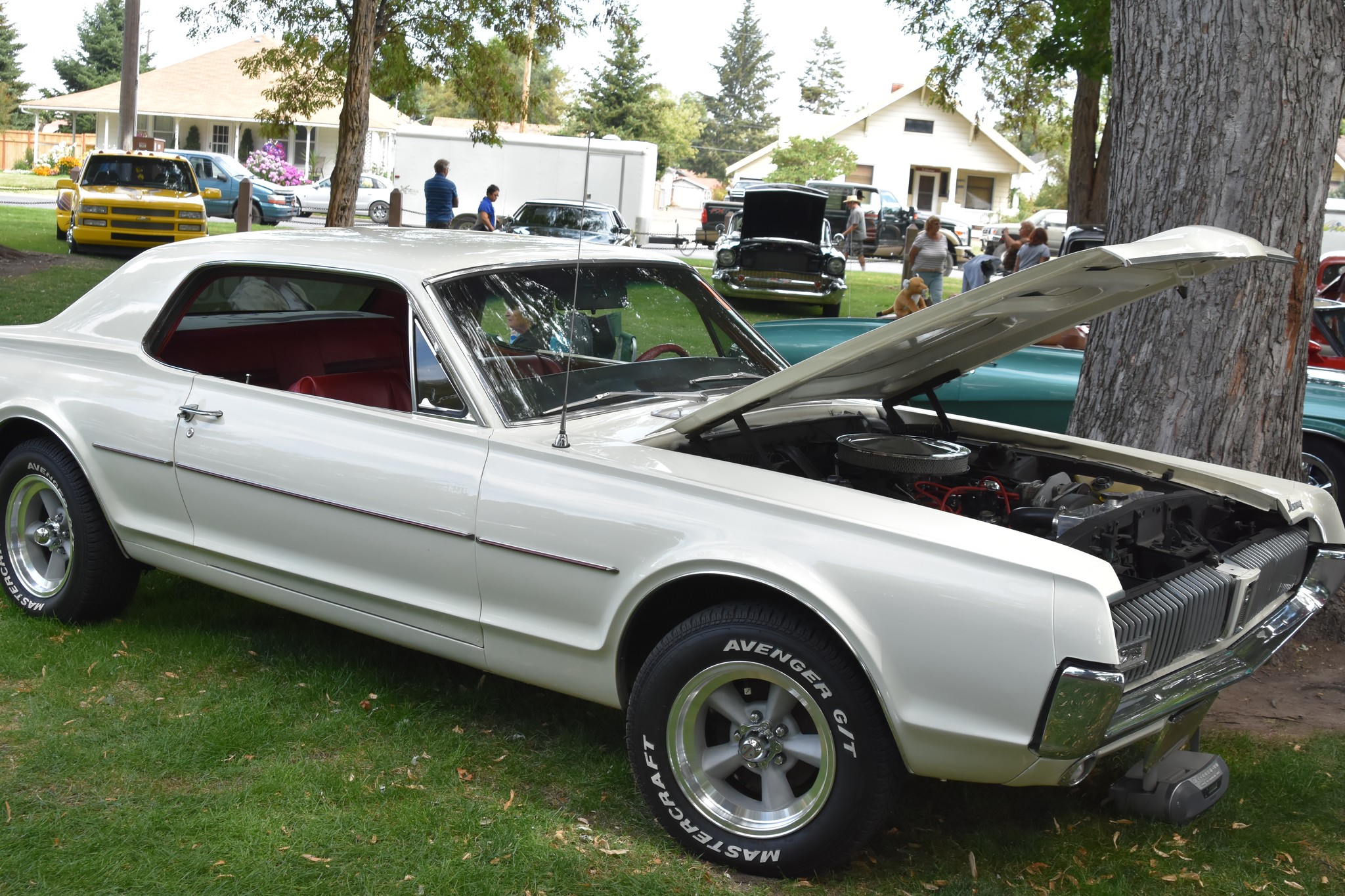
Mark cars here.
[748,296,1345,555]
[2,226,1345,882]
[55,148,222,254]
[488,200,637,249]
[282,172,394,224]
[711,183,848,317]
[978,197,1345,381]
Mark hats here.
[857,191,865,198]
[843,195,861,203]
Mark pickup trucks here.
[694,179,961,278]
[162,148,300,226]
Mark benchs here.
[163,317,410,392]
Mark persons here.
[505,290,565,349]
[424,160,458,229]
[909,216,948,305]
[962,254,1002,293]
[1002,219,1034,276]
[842,195,867,271]
[473,184,501,231]
[1014,227,1050,272]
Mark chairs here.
[289,372,412,413]
[479,355,568,398]
[195,164,208,179]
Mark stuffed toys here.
[876,273,929,318]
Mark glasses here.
[492,193,499,197]
[447,168,450,171]
